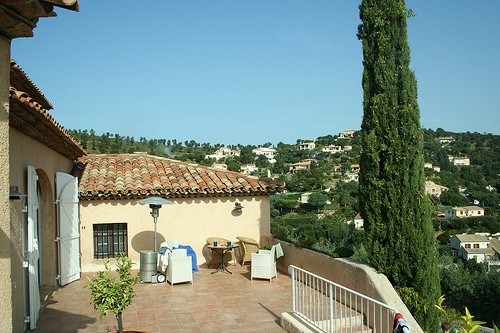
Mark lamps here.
[234,204,243,211]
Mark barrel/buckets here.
[139,250,157,283]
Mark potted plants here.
[81,252,148,333]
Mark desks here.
[207,244,239,274]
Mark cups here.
[227,242,231,246]
[214,242,217,246]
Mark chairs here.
[250,243,285,284]
[206,237,231,268]
[158,249,193,287]
[236,235,259,266]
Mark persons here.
[441,322,451,333]
